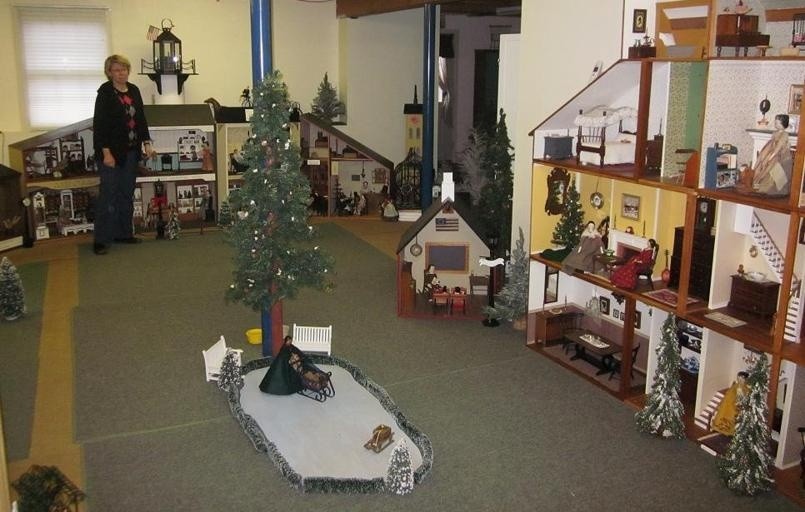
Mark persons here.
[197,141,214,172]
[234,150,244,166]
[359,182,370,196]
[300,138,310,160]
[560,217,611,276]
[93,55,153,255]
[61,209,72,226]
[380,196,397,221]
[349,191,367,216]
[145,200,182,239]
[752,114,793,197]
[200,190,213,219]
[711,371,751,436]
[49,150,71,173]
[611,239,659,290]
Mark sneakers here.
[113,236,142,244]
[93,243,105,254]
